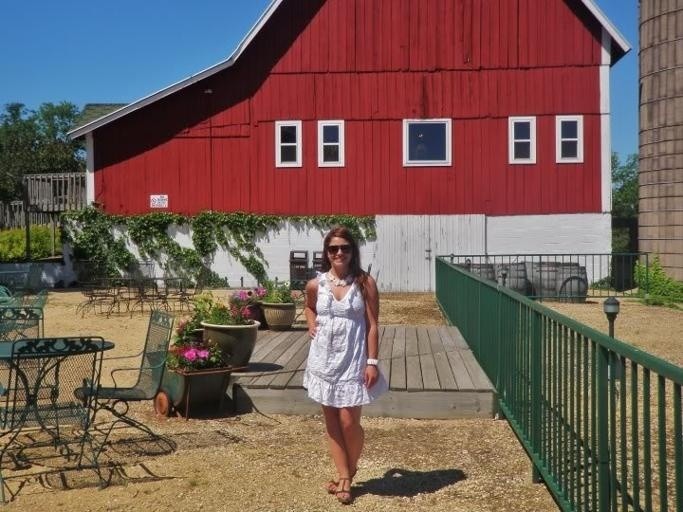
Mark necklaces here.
[327,269,354,286]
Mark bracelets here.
[366,359,378,365]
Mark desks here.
[1,336,115,457]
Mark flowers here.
[162,284,299,370]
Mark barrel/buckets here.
[453,262,588,303]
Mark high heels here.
[336,476,353,503]
[328,467,358,494]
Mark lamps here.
[601,296,620,341]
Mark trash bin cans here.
[289,250,308,290]
[312,251,329,280]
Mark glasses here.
[325,244,353,254]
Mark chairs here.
[74,274,204,320]
[1,335,105,493]
[0,305,65,456]
[1,273,49,341]
[71,307,175,465]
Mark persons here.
[303,227,388,505]
